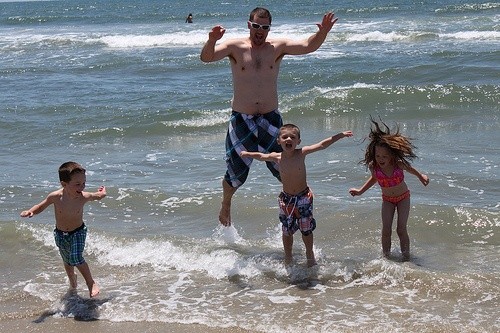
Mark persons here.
[186,13,194,24]
[349,114,429,261]
[20,162,107,298]
[239,123,354,265]
[200,7,339,227]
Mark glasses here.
[251,22,270,31]
